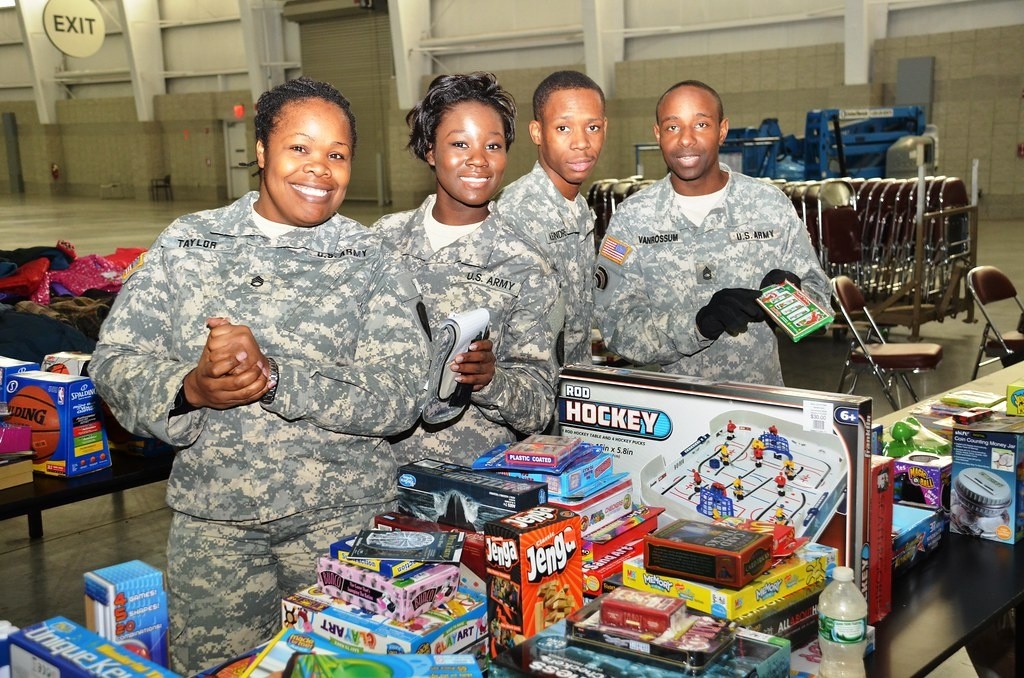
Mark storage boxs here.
[0,351,1024,678]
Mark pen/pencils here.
[449,329,490,408]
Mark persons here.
[369,71,565,466]
[595,79,833,386]
[490,71,609,436]
[87,76,434,676]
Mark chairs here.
[588,171,1024,411]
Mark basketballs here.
[2,386,61,464]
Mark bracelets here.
[262,358,277,402]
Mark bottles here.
[815,565,866,678]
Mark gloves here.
[759,269,803,295]
[696,286,764,339]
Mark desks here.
[0,449,173,545]
[873,362,1024,678]
